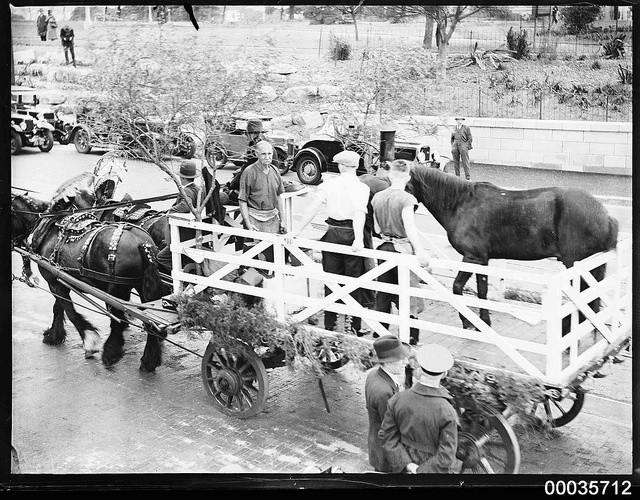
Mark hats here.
[241,120,268,132]
[370,336,413,363]
[332,151,360,167]
[172,161,200,178]
[454,113,465,120]
[416,344,453,378]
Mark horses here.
[8,188,166,373]
[403,164,618,351]
[55,188,198,294]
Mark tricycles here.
[202,114,289,176]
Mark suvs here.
[11,85,55,155]
[73,101,195,159]
[293,140,440,185]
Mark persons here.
[370,157,430,347]
[450,113,474,180]
[377,342,462,473]
[155,159,206,275]
[224,118,280,206]
[281,150,373,338]
[359,174,391,310]
[60,25,77,69]
[45,9,58,42]
[238,140,287,277]
[364,333,412,473]
[36,8,47,41]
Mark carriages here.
[13,206,632,473]
